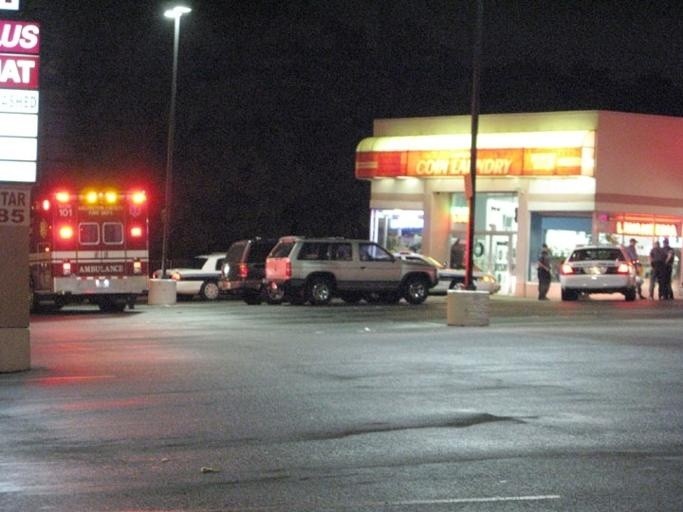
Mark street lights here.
[160,5,193,279]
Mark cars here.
[558,243,637,302]
[376,251,500,296]
[153,251,227,302]
[265,235,440,306]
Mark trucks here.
[28,186,151,314]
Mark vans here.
[218,235,278,305]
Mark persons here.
[534,243,552,300]
[660,237,674,300]
[647,241,668,300]
[625,238,646,300]
